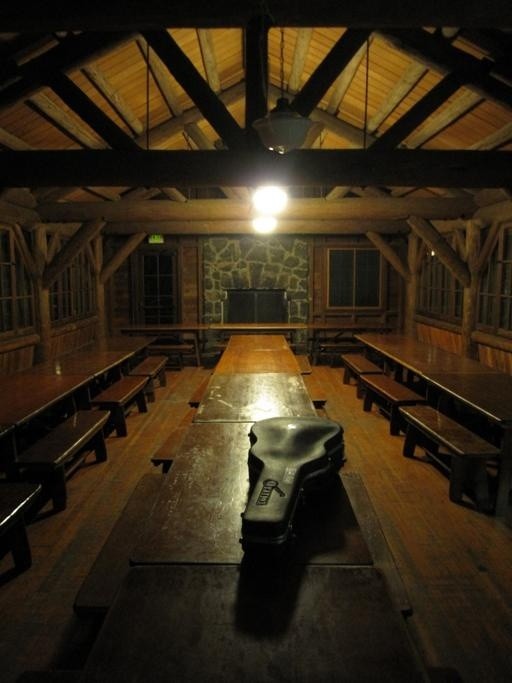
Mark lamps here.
[251,26,315,154]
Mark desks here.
[353,332,511,517]
[111,321,392,362]
[80,565,426,683]
[78,334,159,361]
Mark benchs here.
[0,354,414,613]
